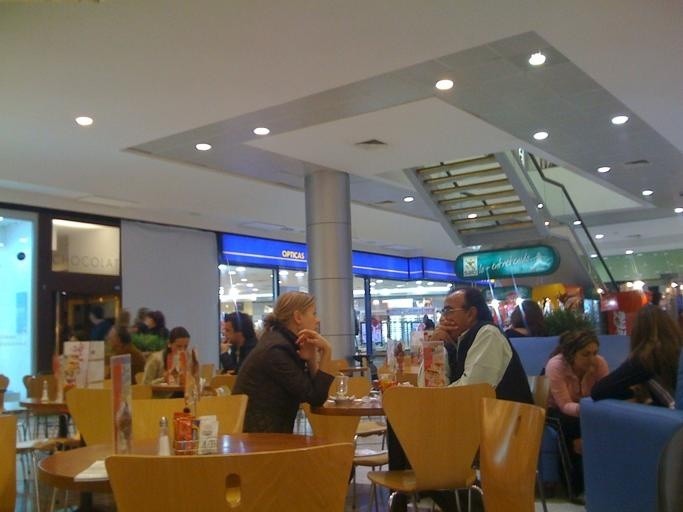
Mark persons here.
[590,304,682,408]
[230,290,337,432]
[503,299,545,338]
[414,287,533,405]
[218,310,258,373]
[59,299,196,399]
[538,328,609,469]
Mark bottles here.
[40,380,49,402]
[152,416,173,456]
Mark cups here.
[333,375,348,397]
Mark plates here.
[329,394,356,403]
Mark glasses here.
[441,308,468,317]
